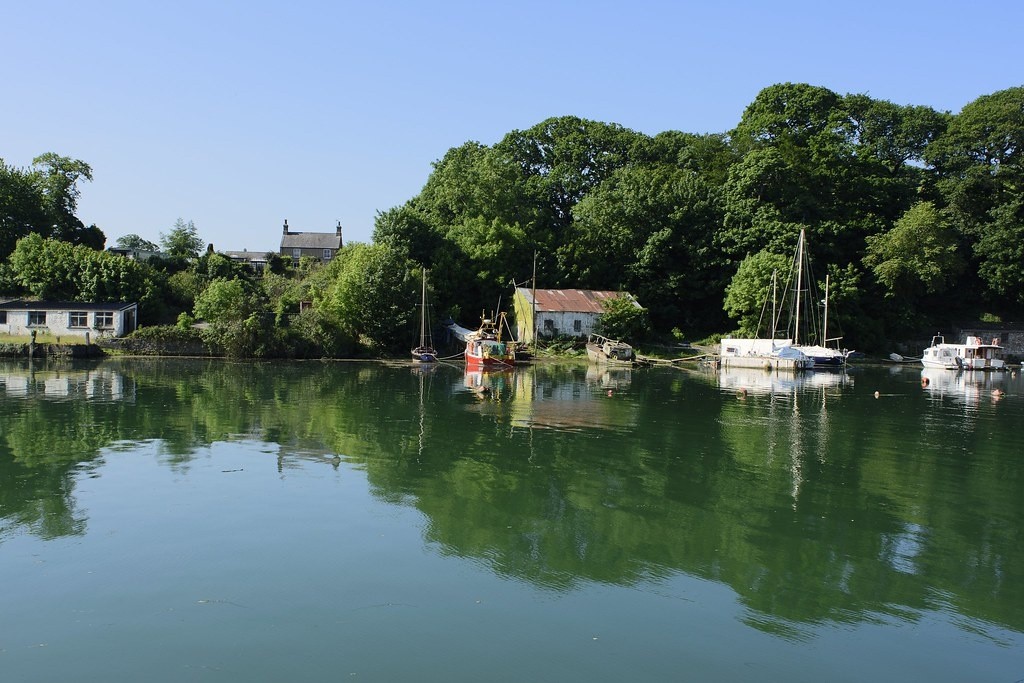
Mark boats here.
[920,333,1008,370]
[463,293,515,368]
[585,333,649,370]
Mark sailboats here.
[720,228,849,370]
[409,267,439,364]
